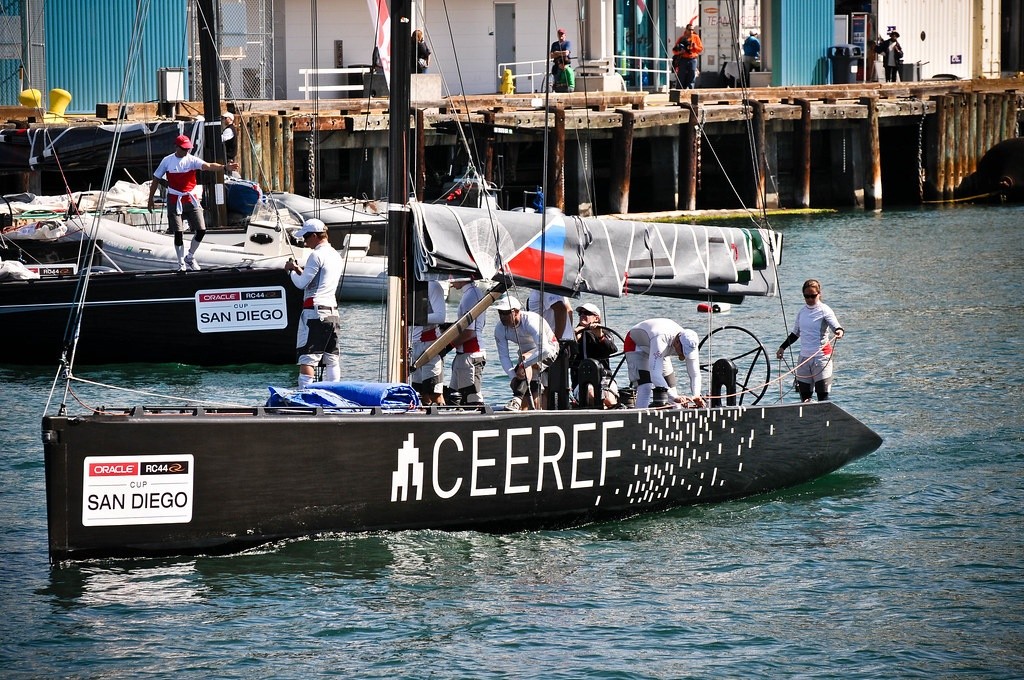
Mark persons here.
[527,284,576,409]
[554,57,575,92]
[777,280,845,400]
[671,24,704,89]
[147,136,239,273]
[625,317,707,411]
[550,28,572,65]
[448,273,487,404]
[570,304,619,407]
[493,296,561,409]
[406,278,447,405]
[742,29,761,81]
[879,31,904,82]
[0,258,39,279]
[284,218,347,388]
[411,30,431,74]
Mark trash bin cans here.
[828,44,862,85]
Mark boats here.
[1,1,506,340]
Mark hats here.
[176,134,194,149]
[294,218,328,237]
[558,28,565,34]
[679,329,699,360]
[576,303,601,318]
[497,295,522,312]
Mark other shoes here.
[177,262,186,271]
[184,257,201,270]
[506,396,521,410]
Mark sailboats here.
[38,1,890,563]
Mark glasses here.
[498,309,514,315]
[687,28,693,31]
[578,310,592,316]
[804,292,819,298]
